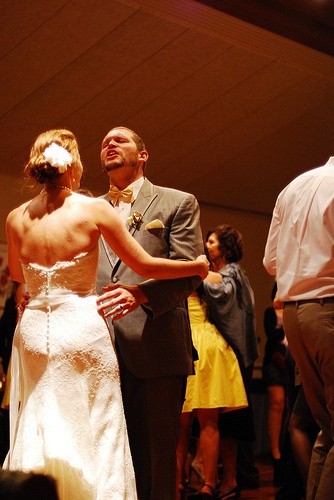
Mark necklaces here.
[38,184,73,194]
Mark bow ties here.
[108,184,133,207]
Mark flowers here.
[43,142,74,174]
[127,210,143,227]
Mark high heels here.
[217,485,240,500]
[187,483,215,500]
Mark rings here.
[119,305,123,310]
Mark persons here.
[289,365,321,474]
[263,154,334,500]
[0,128,209,499]
[264,283,297,468]
[177,283,248,499]
[192,223,261,489]
[13,126,207,500]
